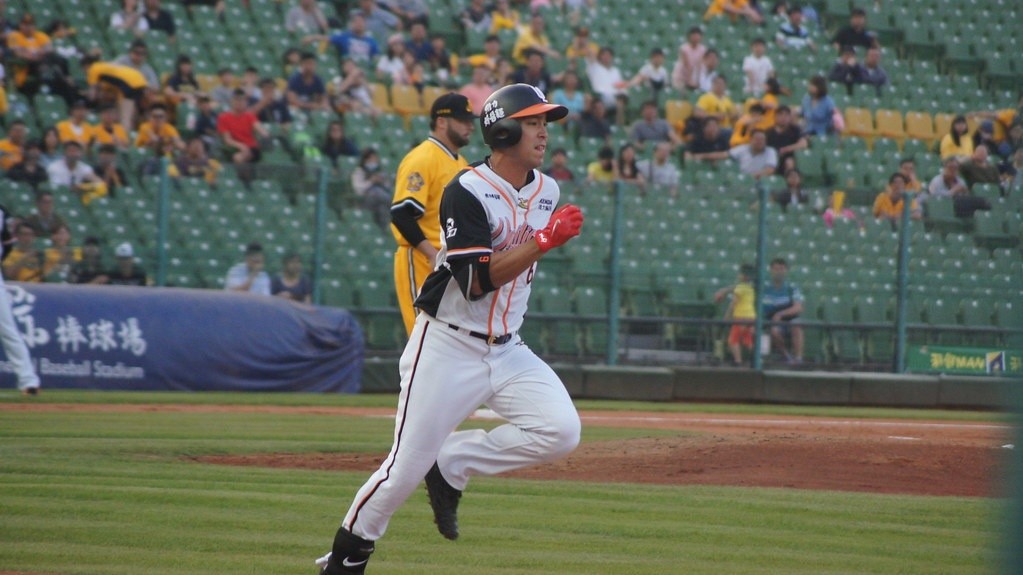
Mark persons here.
[314,84,584,575]
[0,0,1023,395]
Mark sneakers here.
[424,459,462,540]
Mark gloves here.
[535,204,584,254]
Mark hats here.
[431,92,480,119]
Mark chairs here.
[0,1,1022,367]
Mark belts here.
[449,324,517,344]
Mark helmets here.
[479,83,568,147]
